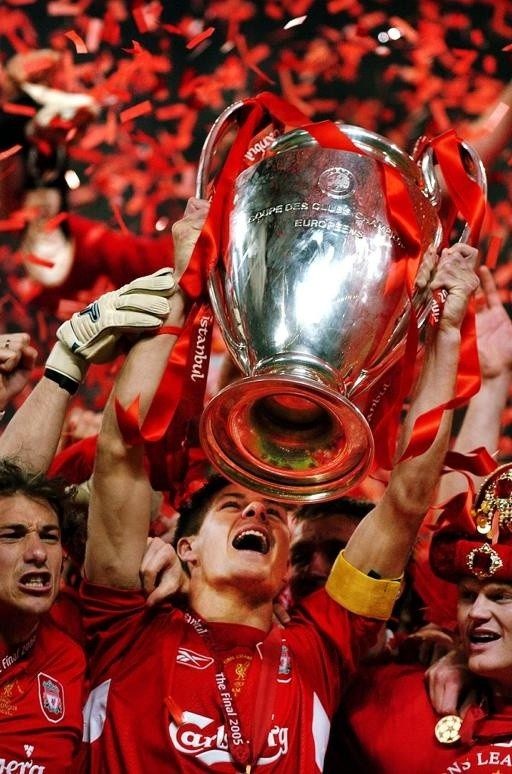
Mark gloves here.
[44,267,175,384]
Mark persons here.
[0,39,510,773]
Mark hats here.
[430,462,512,581]
